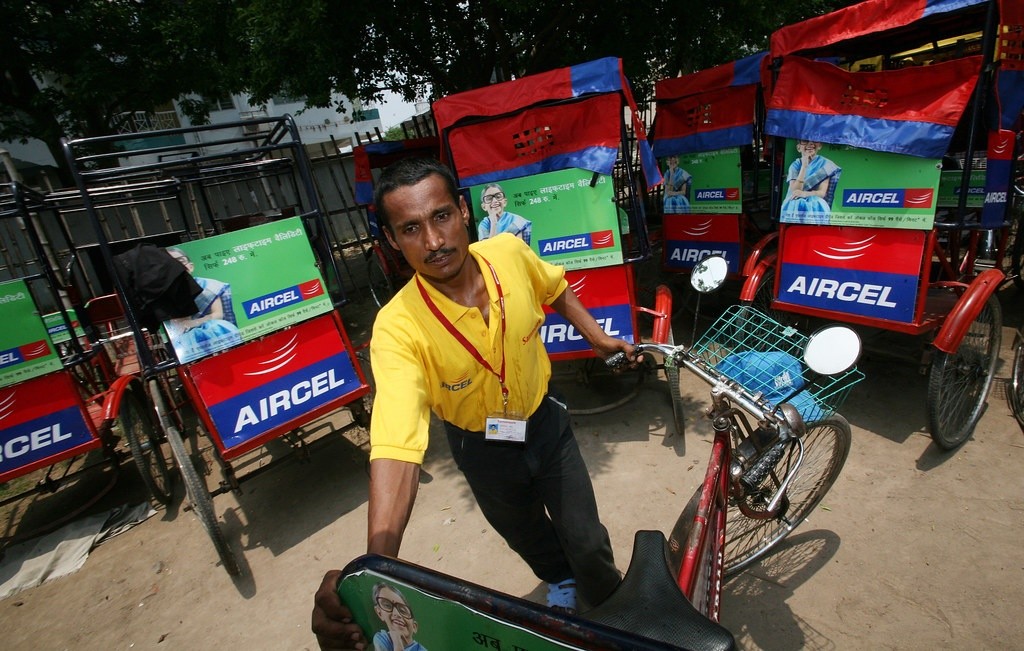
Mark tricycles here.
[62,112,377,579]
[651,46,779,292]
[336,304,867,651]
[763,0,1024,445]
[0,147,171,552]
[351,53,685,440]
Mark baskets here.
[686,306,864,420]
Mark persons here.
[163,248,239,363]
[779,139,842,224]
[662,156,693,213]
[372,582,426,651]
[476,183,532,241]
[312,159,645,651]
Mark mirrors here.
[691,257,728,293]
[804,325,859,375]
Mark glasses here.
[483,192,506,205]
[801,139,818,144]
[667,156,679,161]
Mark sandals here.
[547,577,578,615]
